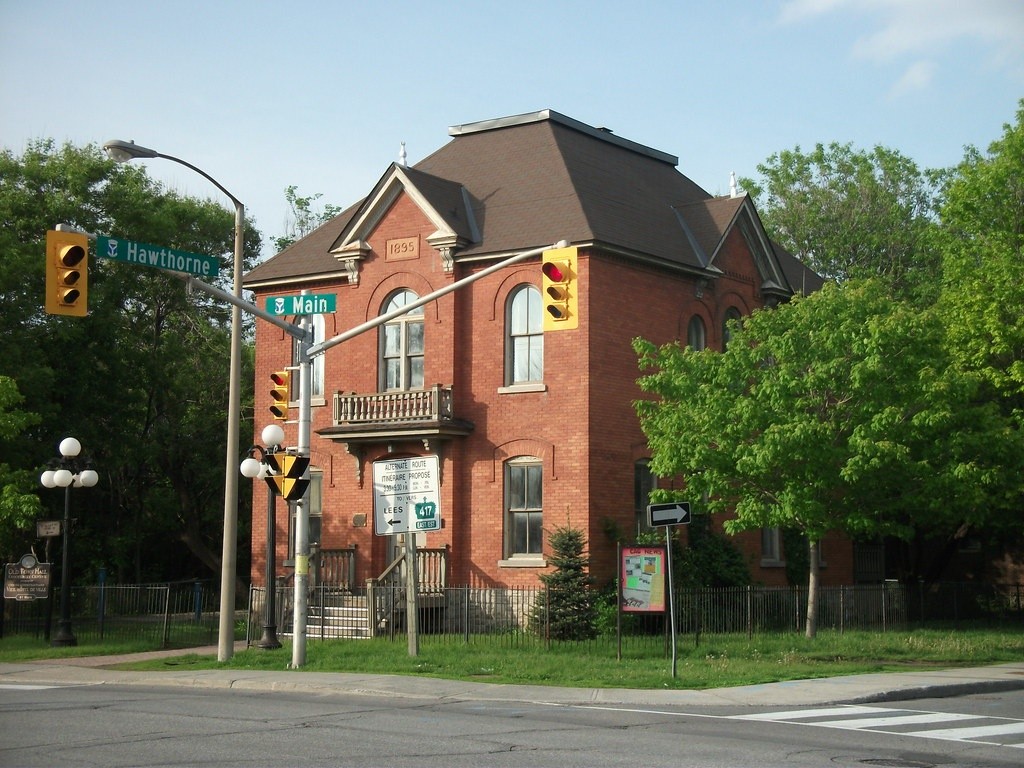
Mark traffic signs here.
[371,454,441,535]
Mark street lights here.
[240,426,298,650]
[42,437,98,649]
[104,138,244,663]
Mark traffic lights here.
[264,453,284,498]
[47,230,89,317]
[270,370,288,420]
[542,248,579,331]
[284,454,311,501]
